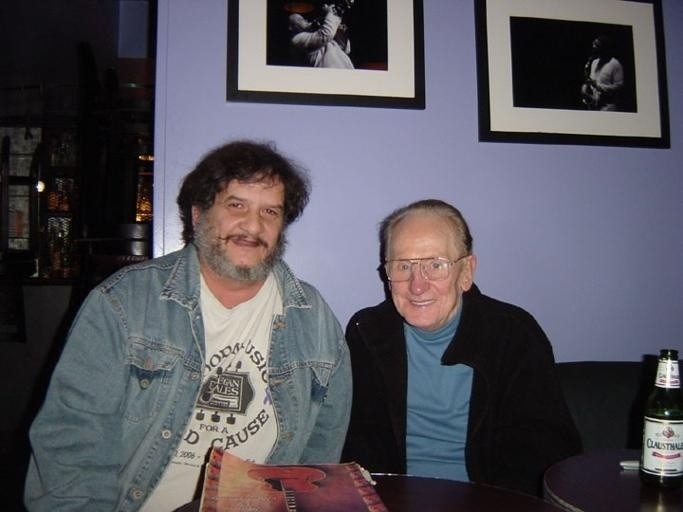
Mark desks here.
[174,472,572,512]
[543,449,683,512]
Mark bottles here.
[638,346,683,490]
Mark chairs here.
[548,360,683,462]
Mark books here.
[195,445,390,512]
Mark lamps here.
[25,125,33,140]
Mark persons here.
[284,1,360,69]
[574,24,623,112]
[338,198,583,500]
[18,139,356,511]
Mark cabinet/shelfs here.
[28,106,154,279]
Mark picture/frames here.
[226,0,426,110]
[472,0,671,149]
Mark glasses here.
[382,255,470,282]
